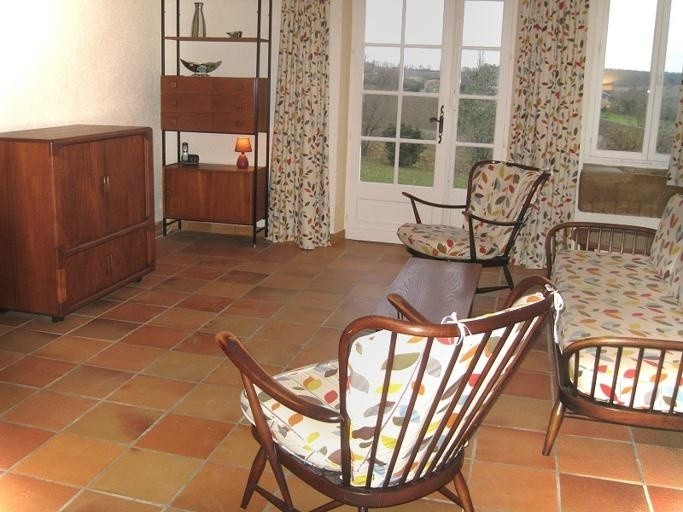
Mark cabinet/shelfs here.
[0,125,155,324]
[160,0,271,248]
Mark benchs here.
[541,193,683,457]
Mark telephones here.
[181,142,199,165]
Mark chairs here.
[214,276,560,512]
[397,160,550,295]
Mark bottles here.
[192,1,205,38]
